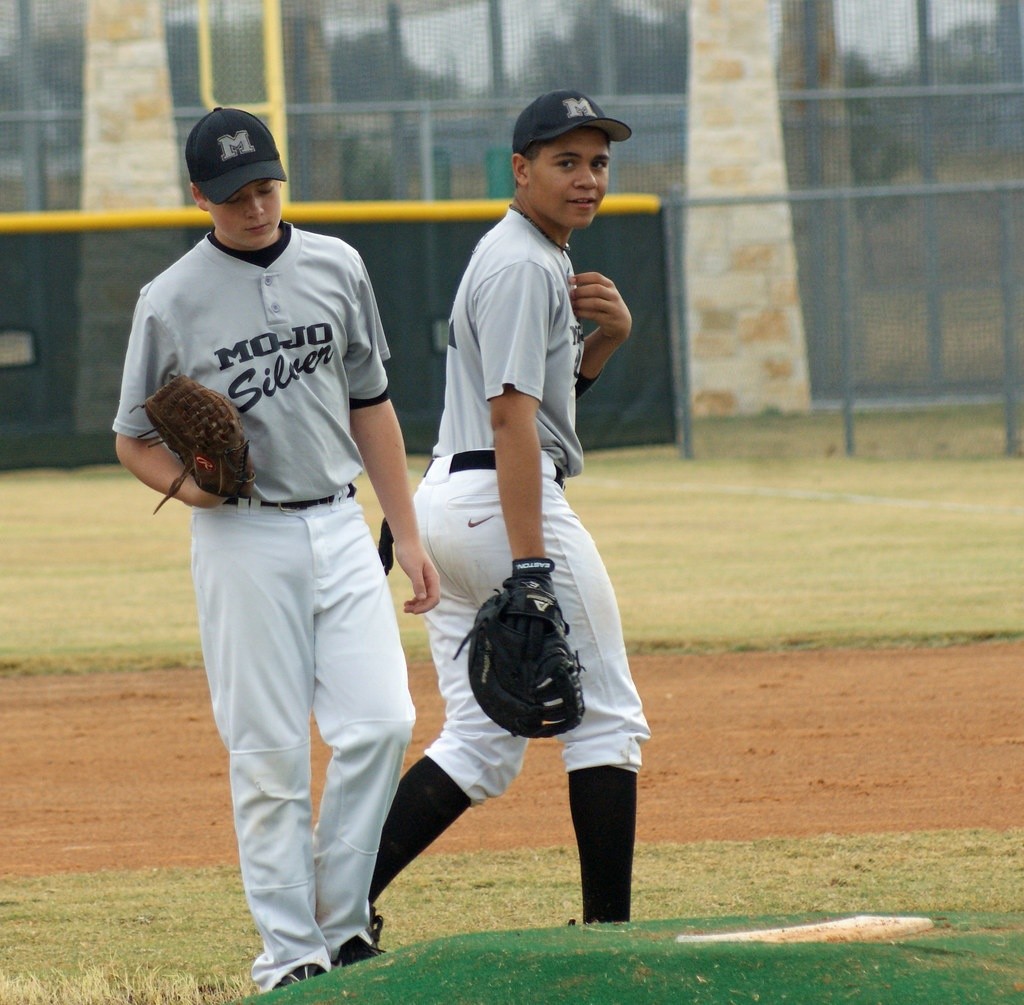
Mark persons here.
[111,104,442,988]
[357,90,654,939]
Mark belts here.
[423,449,565,488]
[223,482,357,510]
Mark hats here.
[511,90,632,155]
[184,106,287,204]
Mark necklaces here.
[509,204,571,250]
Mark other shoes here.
[272,963,324,986]
[329,935,386,967]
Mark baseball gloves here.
[465,575,586,741]
[137,372,258,503]
